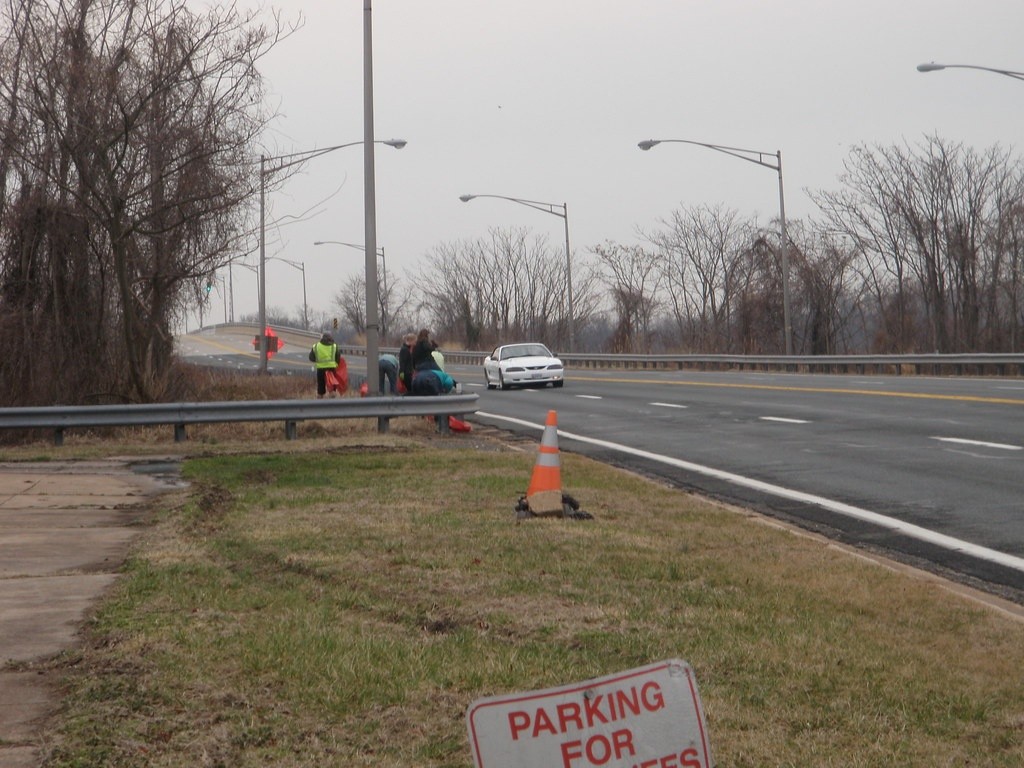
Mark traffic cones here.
[515,408,593,524]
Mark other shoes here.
[331,391,336,398]
[379,392,383,396]
[318,395,323,399]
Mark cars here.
[484,342,564,389]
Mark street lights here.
[638,139,793,355]
[459,194,577,353]
[253,138,406,380]
[262,255,309,332]
[315,241,389,349]
[221,259,260,302]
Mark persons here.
[309,331,341,399]
[378,329,457,424]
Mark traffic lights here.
[207,281,211,291]
